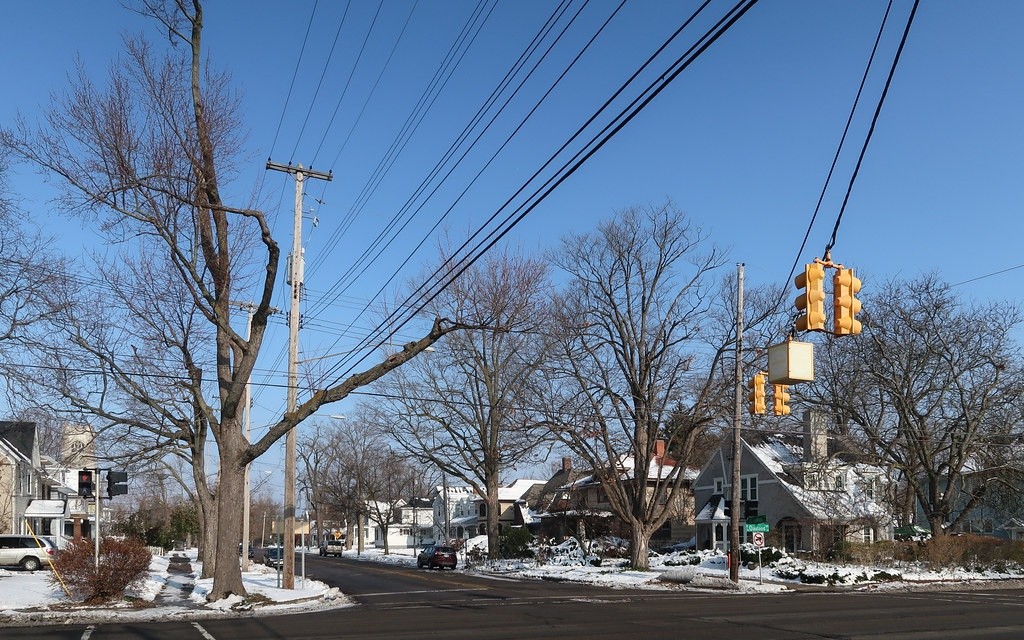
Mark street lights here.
[239,412,346,571]
[281,342,434,590]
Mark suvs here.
[0,533,59,571]
[320,541,342,558]
[417,546,457,570]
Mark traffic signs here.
[745,516,770,532]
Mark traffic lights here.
[772,382,792,416]
[749,374,768,415]
[78,472,91,497]
[833,268,863,337]
[792,263,829,332]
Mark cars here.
[236,544,255,557]
[263,547,285,569]
